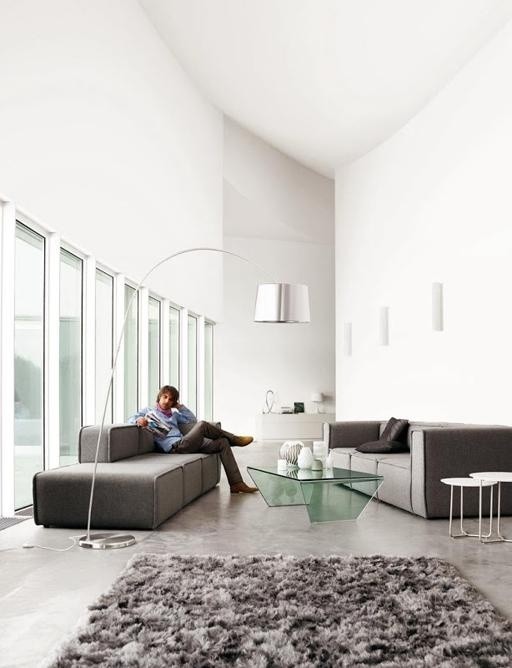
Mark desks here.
[439,471,512,544]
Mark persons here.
[128,384,259,494]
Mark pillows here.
[355,417,409,454]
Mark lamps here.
[76,247,310,549]
[311,392,324,414]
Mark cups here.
[278,457,286,472]
[277,471,286,476]
[312,471,323,479]
[312,459,323,471]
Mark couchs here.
[321,419,512,520]
[32,421,221,530]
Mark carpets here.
[41,552,512,667]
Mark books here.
[140,411,172,439]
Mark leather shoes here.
[231,435,253,447]
[230,482,258,494]
